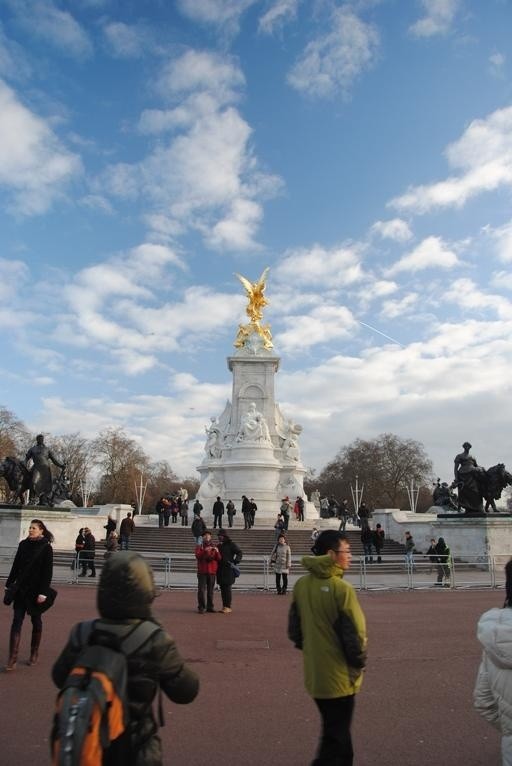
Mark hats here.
[216,530,229,538]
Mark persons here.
[433,482,463,511]
[23,435,64,506]
[284,420,299,447]
[206,416,217,456]
[3,518,58,671]
[240,403,263,439]
[287,530,367,766]
[473,561,512,766]
[49,551,199,766]
[70,495,453,614]
[454,443,477,479]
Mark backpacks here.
[50,617,163,766]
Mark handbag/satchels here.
[271,552,276,563]
[234,567,240,577]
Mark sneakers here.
[219,607,231,613]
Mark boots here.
[26,631,41,665]
[5,632,20,671]
